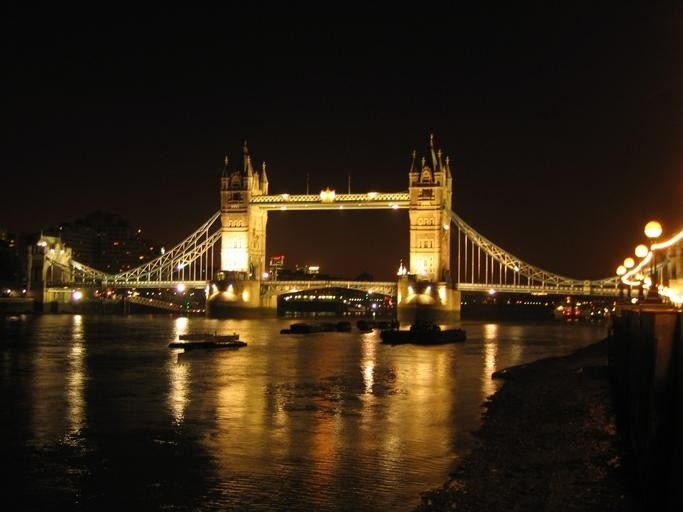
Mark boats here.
[381,321,468,344]
[280,322,352,334]
[357,318,399,332]
[170,330,246,349]
